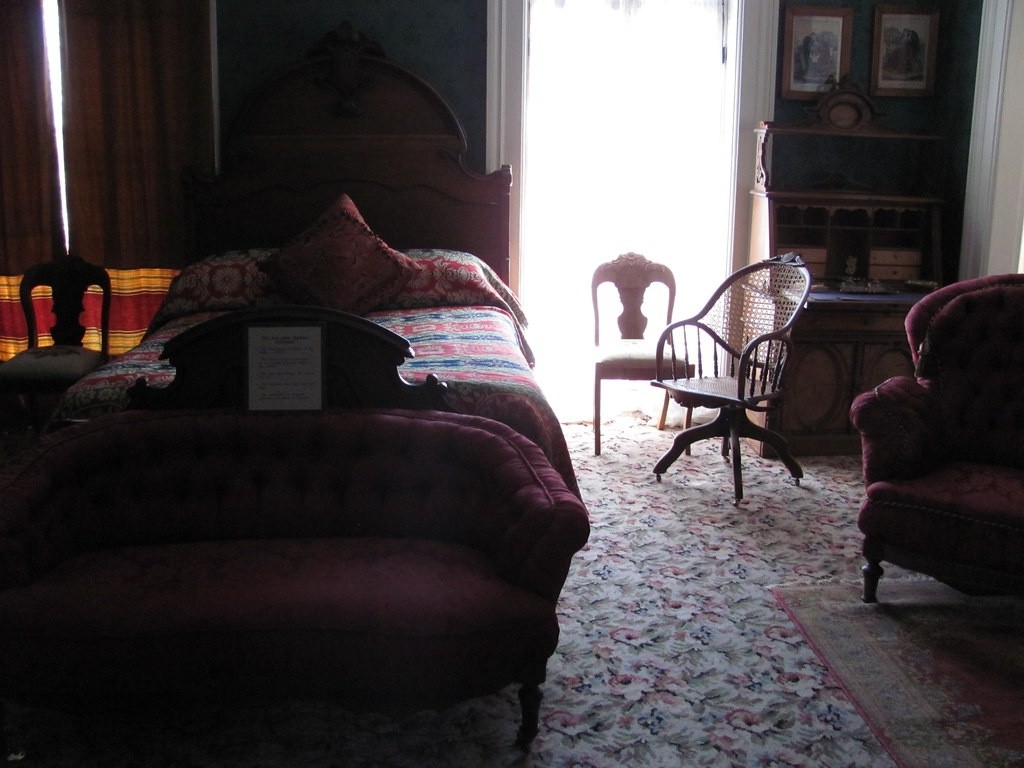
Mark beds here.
[36,135,582,508]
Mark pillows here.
[252,192,414,317]
[368,246,516,314]
[137,247,281,342]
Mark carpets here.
[770,573,1023,768]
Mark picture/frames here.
[869,2,941,101]
[780,3,853,104]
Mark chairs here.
[648,250,812,506]
[590,251,697,458]
[0,253,114,439]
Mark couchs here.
[0,399,595,752]
[847,272,1024,608]
